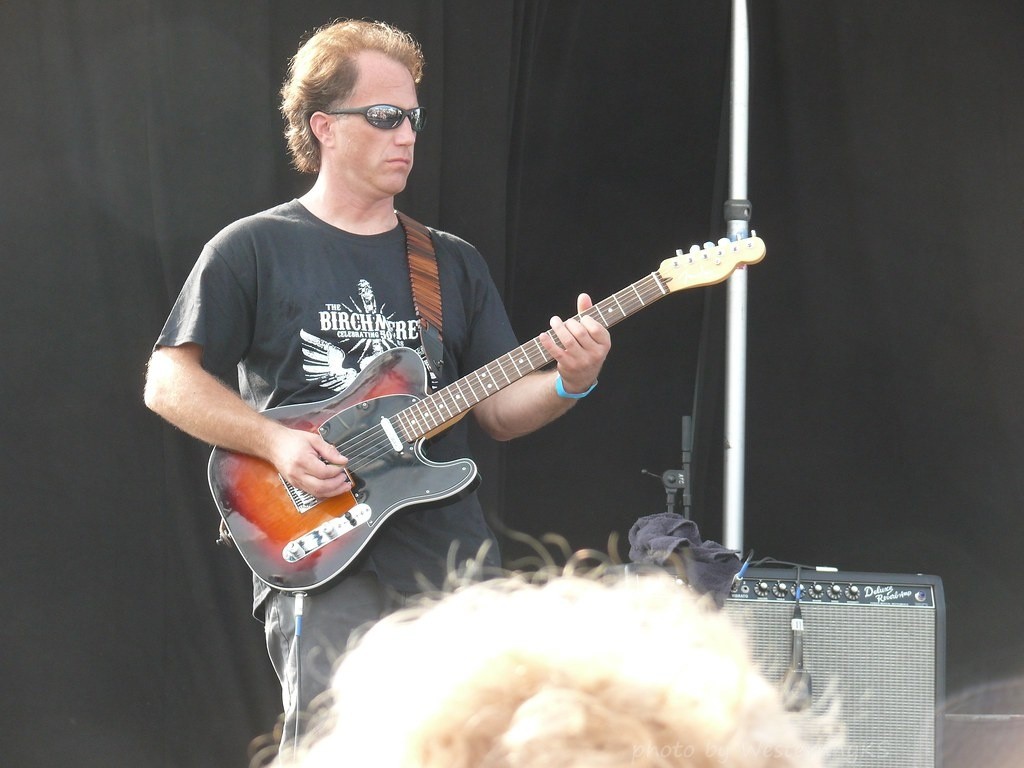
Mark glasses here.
[320,104,427,132]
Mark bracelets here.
[555,374,599,399]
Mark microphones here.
[784,602,804,712]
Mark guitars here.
[207,229,766,593]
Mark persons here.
[143,16,612,763]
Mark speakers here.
[719,567,946,768]
